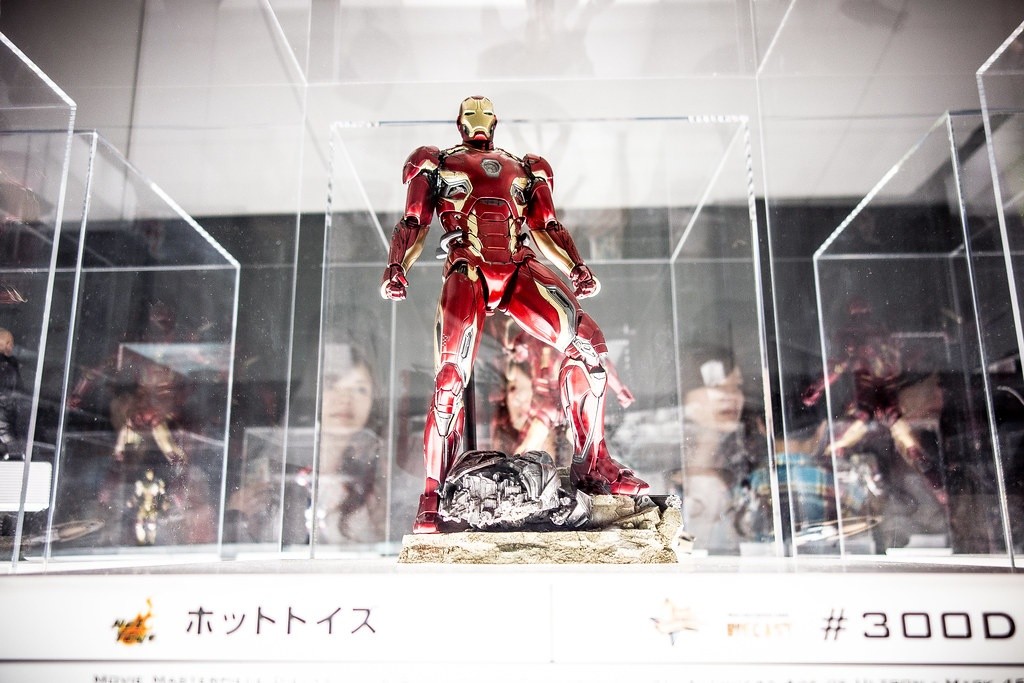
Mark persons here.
[492,348,573,469]
[802,298,976,539]
[221,345,416,545]
[805,343,951,537]
[66,301,228,543]
[634,342,774,543]
[126,468,175,544]
[379,97,648,533]
[723,373,881,542]
